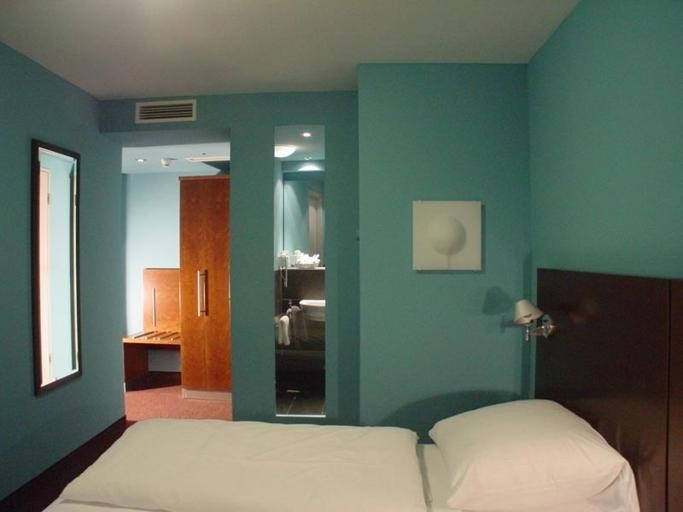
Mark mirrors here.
[283,170,325,266]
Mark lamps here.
[514,299,554,341]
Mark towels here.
[278,306,308,346]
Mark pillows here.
[428,399,641,512]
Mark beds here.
[42,269,683,512]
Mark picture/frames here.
[31,137,82,398]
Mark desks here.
[122,268,181,393]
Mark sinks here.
[299,299,326,322]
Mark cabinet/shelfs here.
[177,175,236,400]
